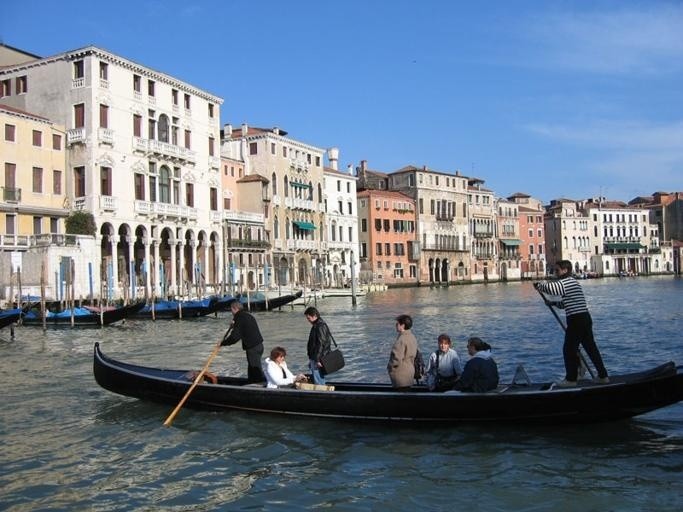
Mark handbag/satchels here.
[435,374,457,392]
[322,349,345,375]
[414,350,425,378]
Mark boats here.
[92,341,683,429]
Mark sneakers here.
[557,379,576,389]
[593,377,608,384]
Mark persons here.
[453,337,499,393]
[387,315,417,391]
[534,260,610,388]
[263,347,307,390]
[425,334,463,392]
[221,301,265,385]
[304,307,332,385]
